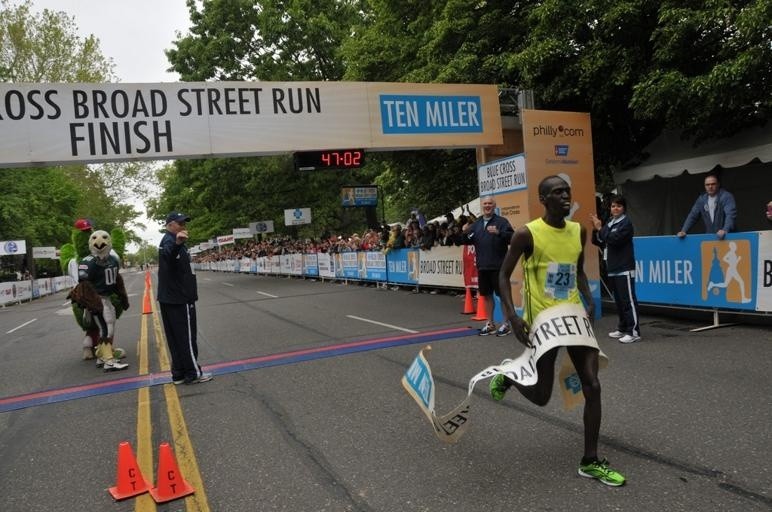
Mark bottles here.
[166,213,191,223]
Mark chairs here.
[608,330,631,338]
[578,455,625,486]
[95,358,129,370]
[479,321,497,336]
[498,322,511,336]
[173,371,213,385]
[489,359,512,401]
[618,335,641,343]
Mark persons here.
[156,212,213,386]
[677,173,737,238]
[191,204,478,301]
[462,198,515,337]
[489,174,626,487]
[591,195,641,343]
[15,269,49,281]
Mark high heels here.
[460,288,476,314]
[147,444,195,505]
[107,440,154,503]
[470,295,488,321]
[143,271,153,314]
